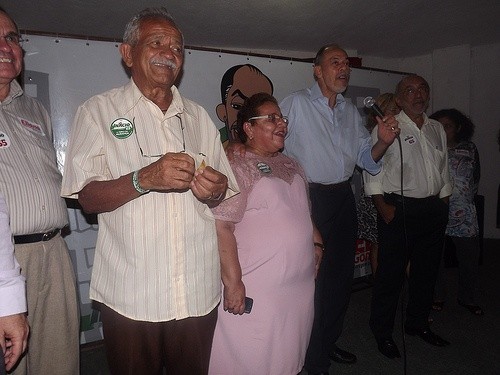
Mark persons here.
[355,92,415,286]
[209,93,327,375]
[0,4,83,375]
[61,5,241,375]
[277,44,403,375]
[0,180,31,375]
[363,74,453,358]
[428,107,486,316]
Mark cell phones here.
[245,297,253,313]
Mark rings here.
[207,192,214,200]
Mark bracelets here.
[134,170,151,194]
[314,242,326,254]
[212,193,224,202]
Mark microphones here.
[364,96,395,130]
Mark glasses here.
[248,115,289,125]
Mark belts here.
[12,227,61,245]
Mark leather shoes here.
[406,323,450,347]
[327,345,357,365]
[377,333,400,360]
